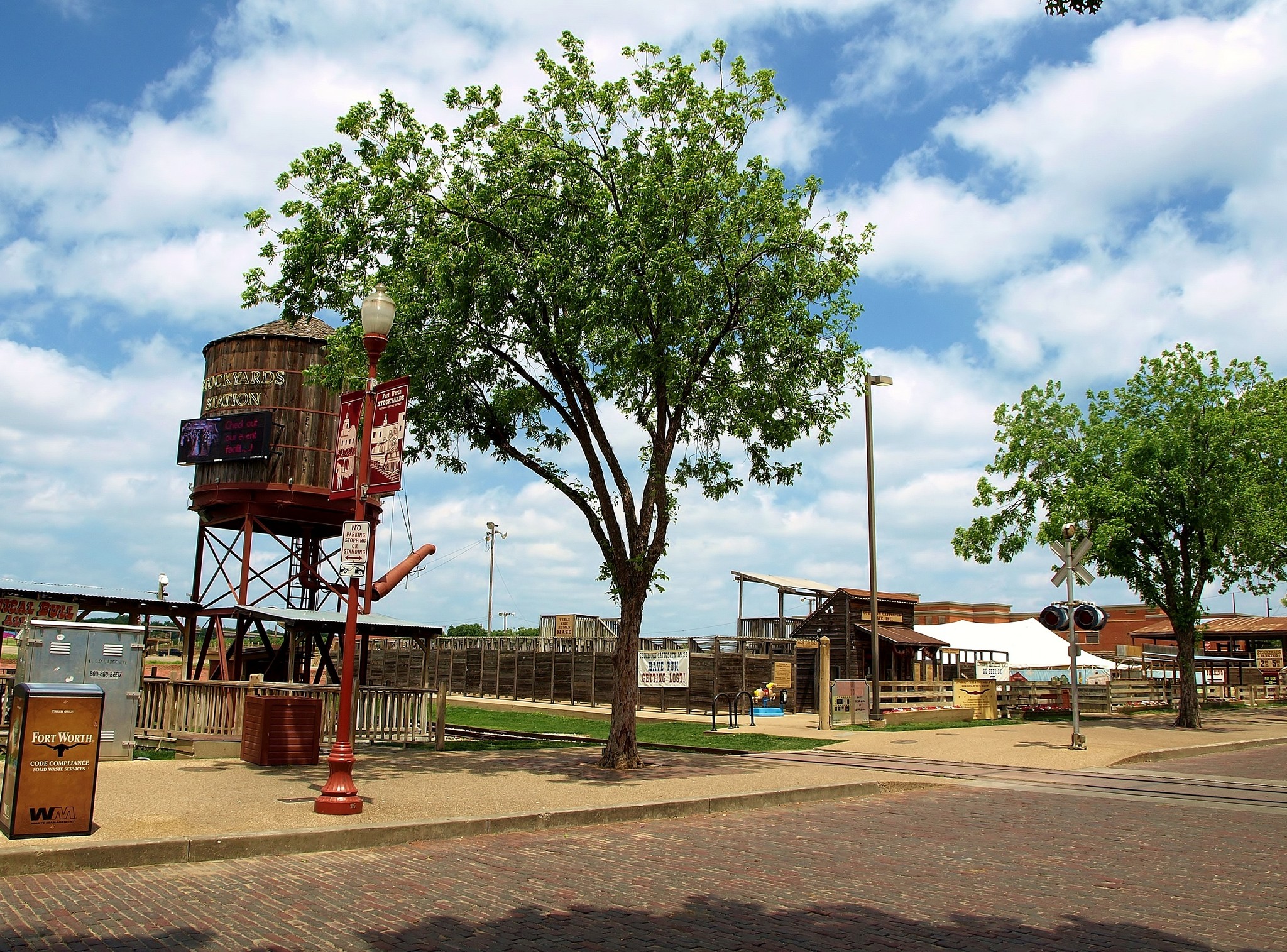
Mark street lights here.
[312,280,398,816]
[864,372,893,727]
[484,521,508,636]
[498,612,515,633]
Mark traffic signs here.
[341,520,369,565]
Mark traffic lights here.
[1039,606,1098,630]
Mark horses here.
[751,682,778,705]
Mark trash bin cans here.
[1040,689,1070,708]
[0,682,106,840]
